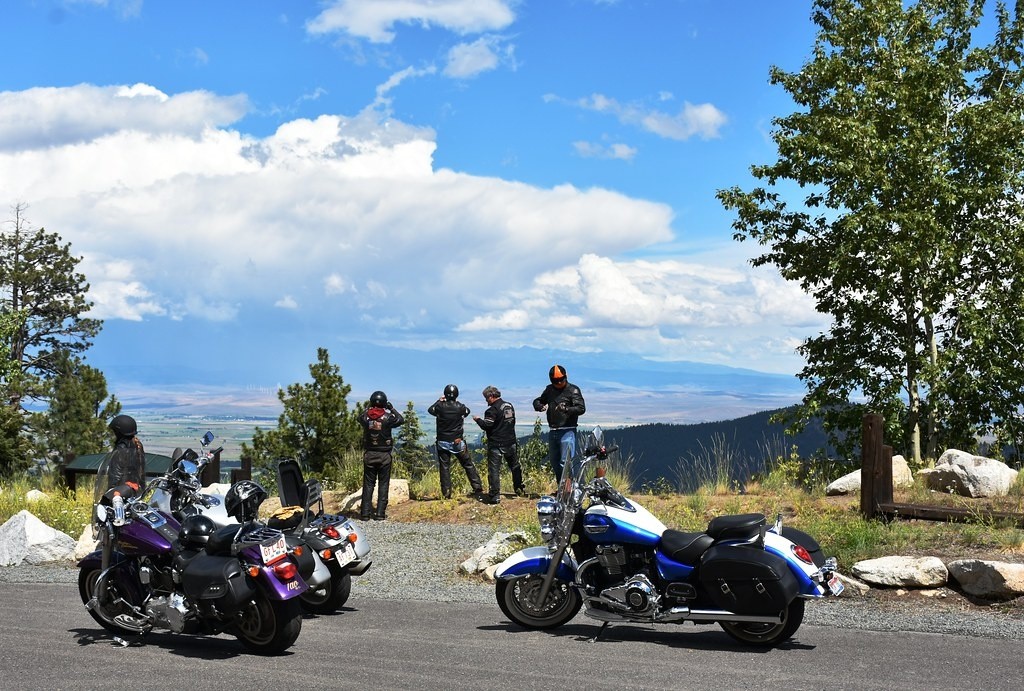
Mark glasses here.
[551,377,566,385]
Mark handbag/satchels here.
[181,555,255,624]
[698,546,801,617]
[781,525,826,570]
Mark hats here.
[549,364,567,384]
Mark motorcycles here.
[496,424,845,649]
[76,430,372,654]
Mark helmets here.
[177,514,218,543]
[109,414,139,446]
[224,479,269,517]
[370,391,387,408]
[444,384,459,400]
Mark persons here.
[473,386,525,505]
[107,415,146,491]
[428,384,486,501]
[356,391,406,521]
[533,365,586,498]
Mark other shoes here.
[483,496,500,504]
[514,485,526,497]
[474,489,484,495]
[361,512,377,521]
[444,495,451,500]
[375,512,389,520]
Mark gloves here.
[385,402,393,410]
[554,401,569,414]
[536,403,548,412]
[365,404,370,409]
